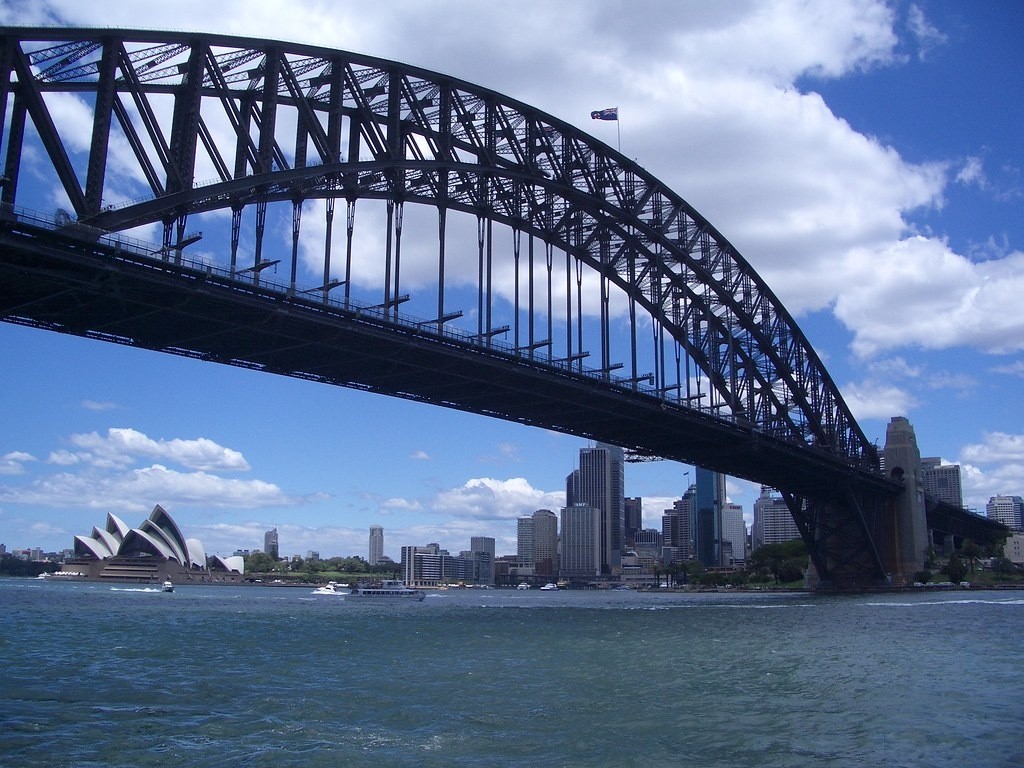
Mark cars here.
[659,582,672,588]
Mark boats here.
[309,584,348,596]
[517,583,531,590]
[540,583,560,591]
[344,579,428,603]
[161,581,175,593]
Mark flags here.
[591,108,617,121]
